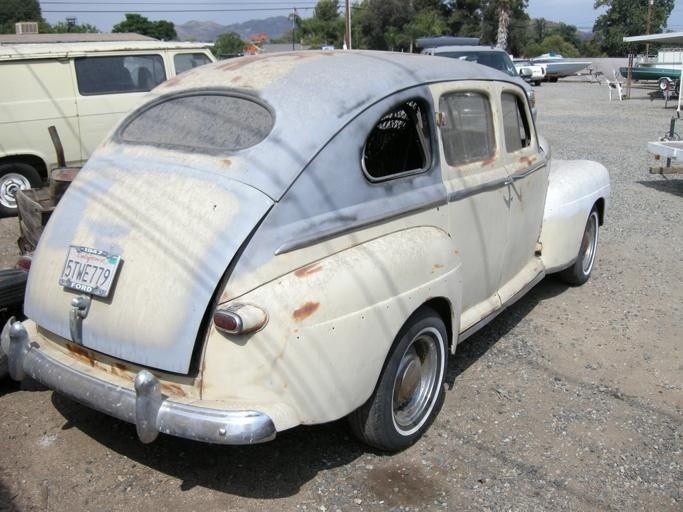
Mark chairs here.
[606,80,623,102]
